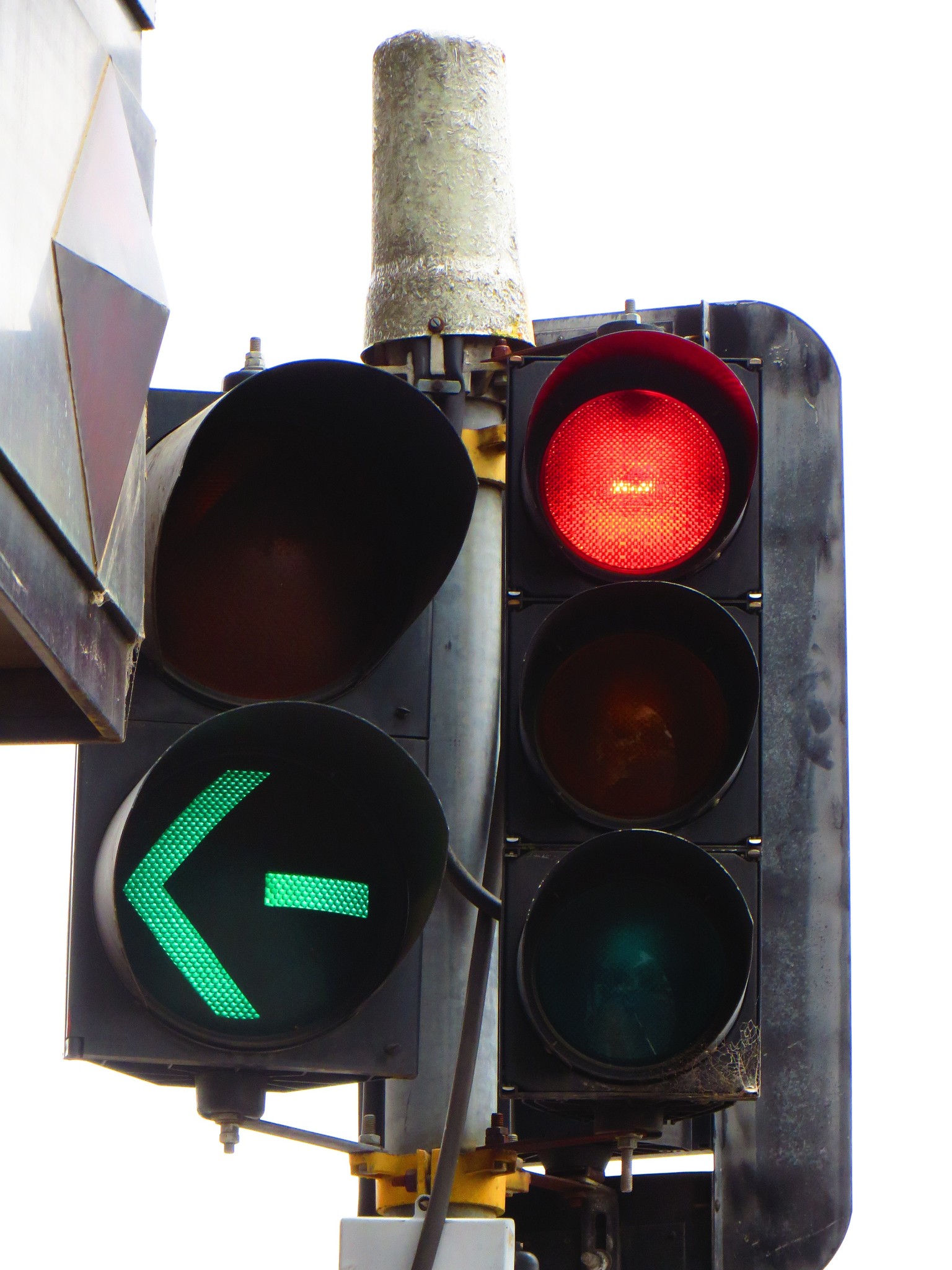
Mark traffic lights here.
[67,358,480,1079]
[495,326,763,1118]
